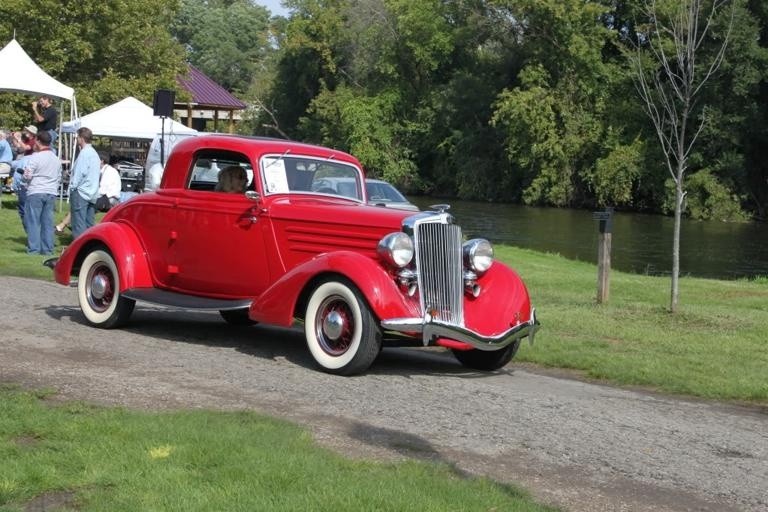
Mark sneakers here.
[55,225,64,236]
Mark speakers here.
[154,88,176,116]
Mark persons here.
[213,166,250,193]
[0,95,122,256]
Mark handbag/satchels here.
[97,194,111,212]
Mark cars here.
[55,131,540,378]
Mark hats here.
[25,125,38,135]
